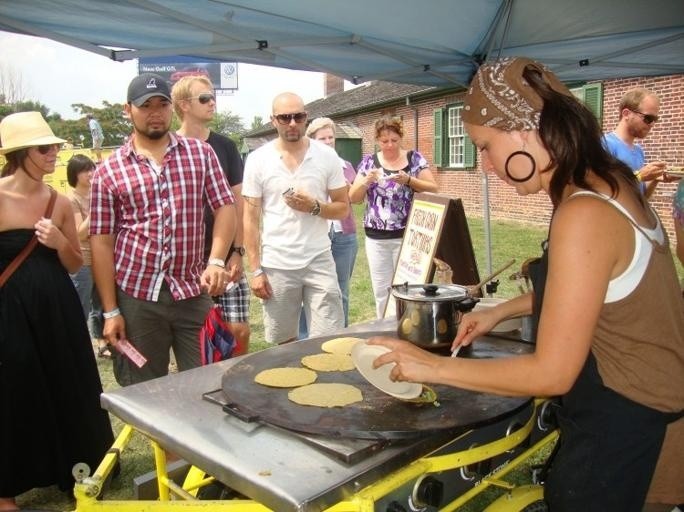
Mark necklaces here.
[71,187,89,200]
[378,147,403,167]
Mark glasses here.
[35,145,51,155]
[273,112,308,125]
[188,93,215,105]
[630,109,659,124]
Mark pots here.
[388,282,479,350]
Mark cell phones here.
[282,186,296,199]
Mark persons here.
[59,154,117,359]
[238,92,352,346]
[344,113,440,319]
[670,179,684,265]
[86,71,239,385]
[86,113,104,164]
[0,109,125,511]
[166,75,252,361]
[363,56,684,472]
[295,116,359,341]
[596,89,684,200]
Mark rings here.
[370,173,373,177]
[223,280,228,285]
[106,339,109,344]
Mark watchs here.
[310,199,321,216]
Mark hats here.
[128,73,173,107]
[1,111,69,154]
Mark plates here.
[352,339,425,400]
[662,167,684,178]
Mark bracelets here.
[233,246,245,255]
[253,269,263,277]
[208,258,226,268]
[633,170,642,181]
[102,308,120,319]
[361,178,369,189]
[407,177,410,183]
